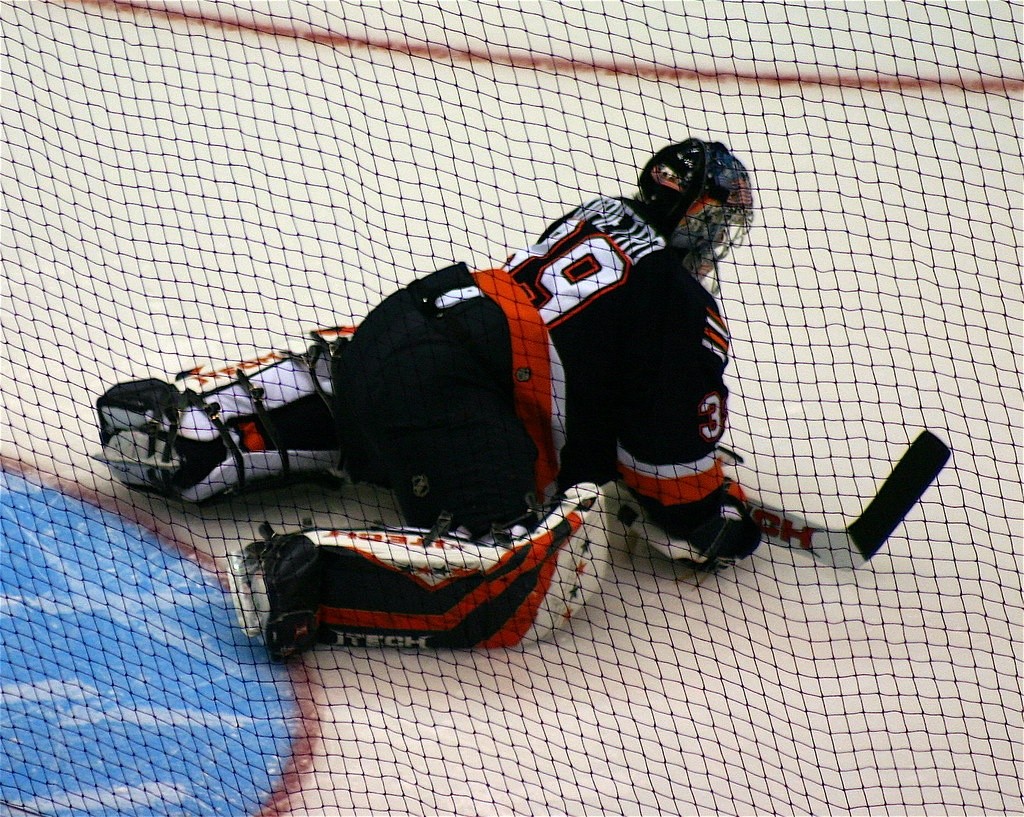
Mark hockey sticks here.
[738,431,950,572]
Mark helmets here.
[638,136,754,281]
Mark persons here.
[96,138,763,664]
